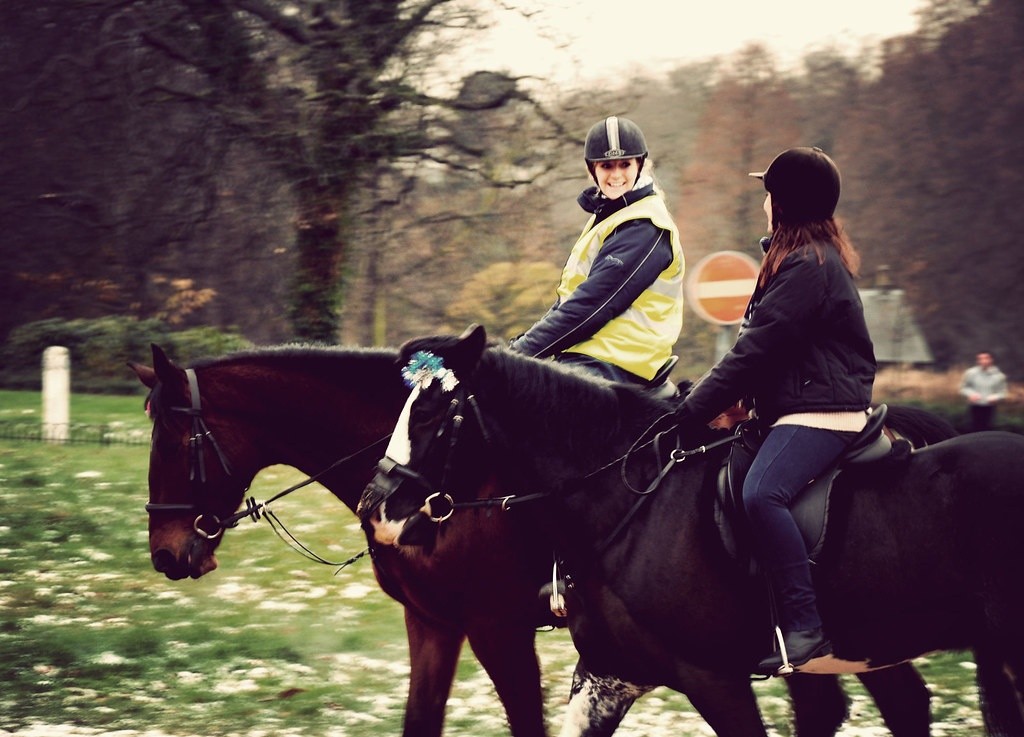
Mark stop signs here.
[689,253,760,326]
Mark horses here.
[125,322,1024,737]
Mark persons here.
[506,116,685,387]
[957,351,1008,435]
[656,145,876,671]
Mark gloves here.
[674,401,697,426]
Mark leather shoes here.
[758,627,828,670]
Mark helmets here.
[749,147,840,219]
[584,116,648,162]
